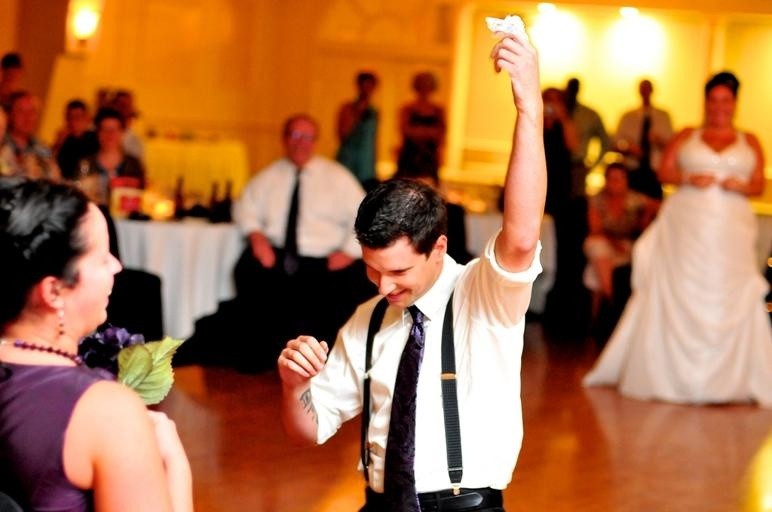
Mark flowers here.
[78,328,184,406]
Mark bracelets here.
[682,172,690,184]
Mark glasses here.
[286,131,315,141]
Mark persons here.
[540,88,586,338]
[0,184,193,512]
[109,90,143,160]
[585,163,661,328]
[563,77,609,199]
[0,51,24,135]
[230,114,367,373]
[585,69,770,405]
[56,100,99,180]
[78,108,145,207]
[0,91,62,179]
[279,14,549,512]
[397,74,445,178]
[615,79,670,201]
[334,73,380,181]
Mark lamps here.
[65,0,102,48]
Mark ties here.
[640,116,652,170]
[383,305,421,512]
[284,169,300,276]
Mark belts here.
[420,492,483,512]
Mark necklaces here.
[1,339,85,363]
